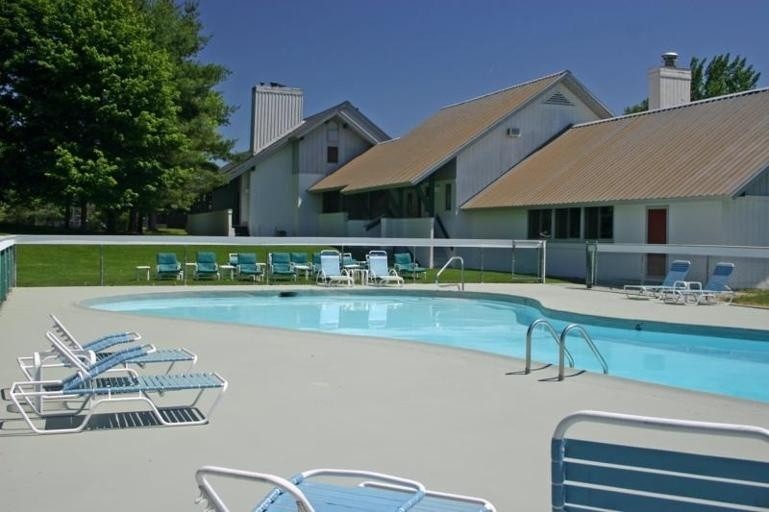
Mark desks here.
[136,266,151,282]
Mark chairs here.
[195,464,497,511]
[9,314,229,435]
[624,260,736,306]
[549,407,768,511]
[156,249,426,286]
